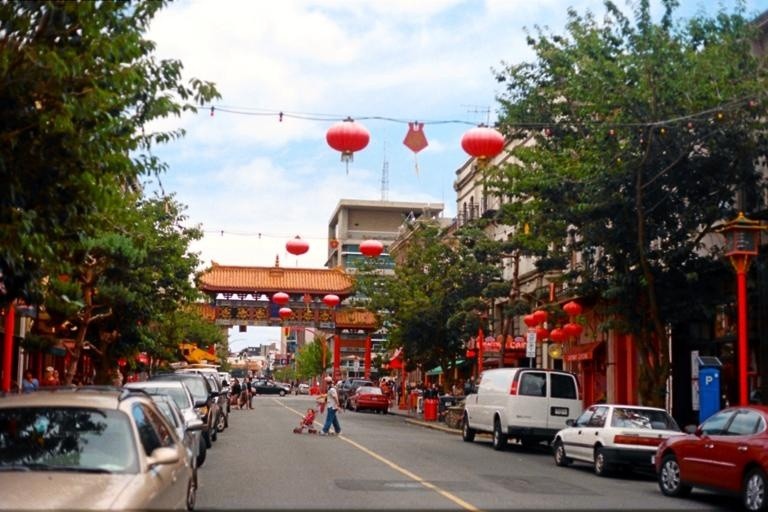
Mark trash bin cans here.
[424,398,437,422]
[439,397,466,423]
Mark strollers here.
[293,411,318,433]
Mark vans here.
[462,367,584,451]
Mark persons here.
[316,375,344,436]
[324,372,334,389]
[299,407,315,427]
[229,378,241,410]
[245,378,254,409]
[239,377,250,410]
[22,369,39,395]
[370,373,477,416]
[41,365,58,387]
[53,368,64,386]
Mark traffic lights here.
[284,325,291,337]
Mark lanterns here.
[522,301,584,349]
[321,293,340,310]
[278,307,293,322]
[460,125,506,172]
[325,119,371,176]
[465,350,476,359]
[358,238,384,260]
[285,238,311,265]
[272,291,290,306]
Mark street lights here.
[714,210,768,405]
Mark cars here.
[0,364,232,512]
[550,403,768,512]
[336,377,389,414]
[250,380,309,397]
